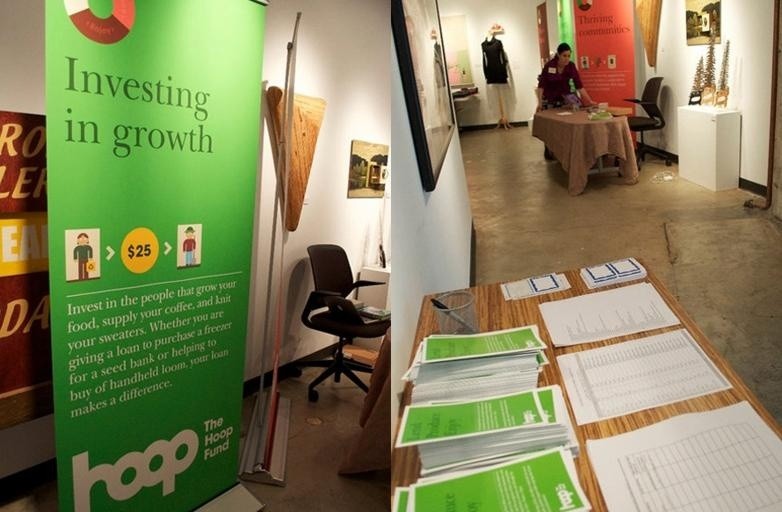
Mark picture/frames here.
[390,0,455,192]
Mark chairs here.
[623,77,672,172]
[291,244,391,403]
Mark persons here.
[478,34,511,87]
[536,44,599,162]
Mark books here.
[391,321,594,512]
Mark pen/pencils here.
[430,296,476,331]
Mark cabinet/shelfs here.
[353,262,391,352]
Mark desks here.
[534,106,628,177]
[390,257,782,511]
